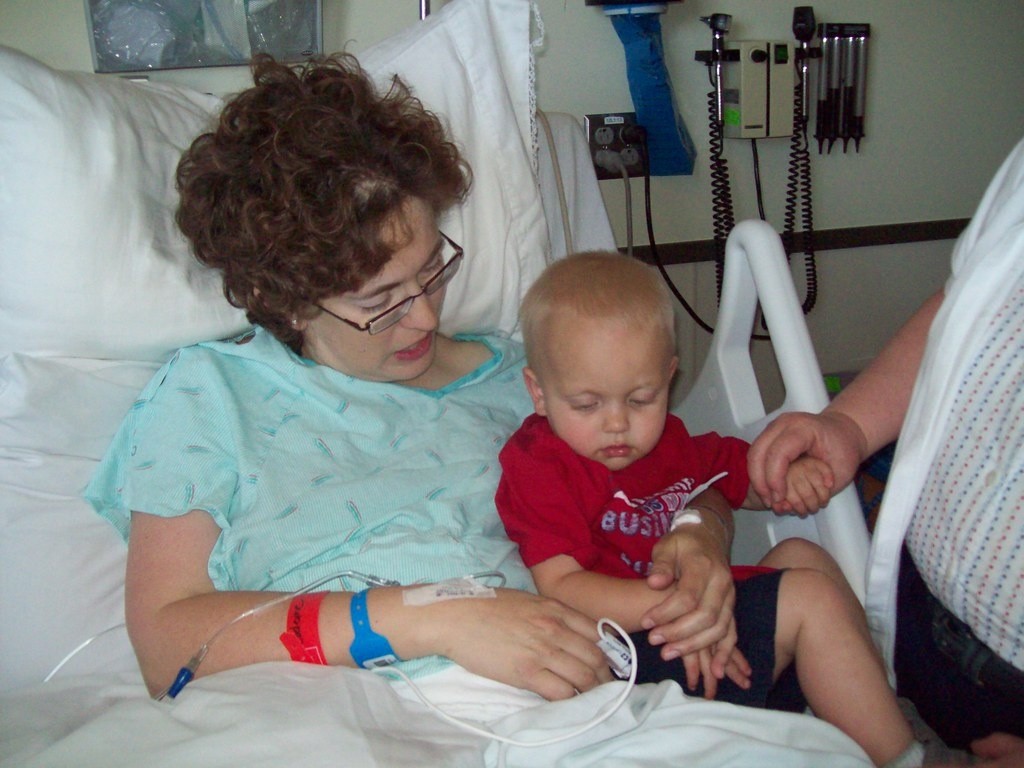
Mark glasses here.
[297,229,462,336]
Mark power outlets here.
[585,113,649,180]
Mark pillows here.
[2,1,550,352]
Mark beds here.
[0,113,867,766]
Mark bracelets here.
[280,589,329,668]
[347,589,405,674]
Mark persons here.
[79,49,744,739]
[490,249,931,768]
[746,130,1024,768]
